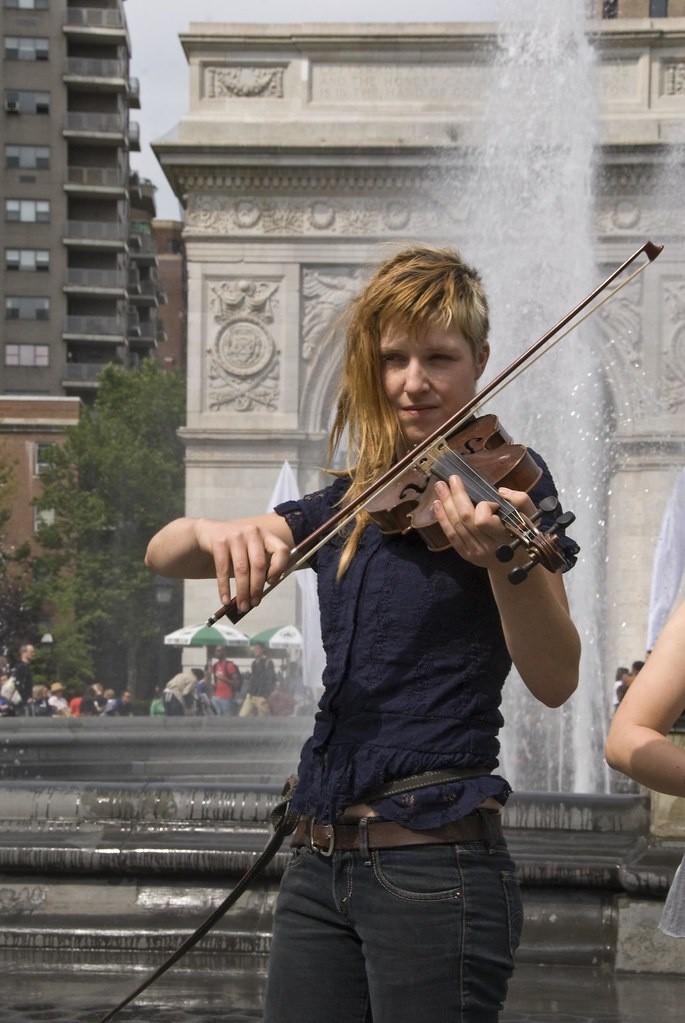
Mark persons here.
[612,649,651,712]
[145,247,584,1023]
[604,597,685,938]
[0,643,314,718]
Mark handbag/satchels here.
[0,664,28,705]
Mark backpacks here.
[213,662,244,691]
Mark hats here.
[51,682,65,693]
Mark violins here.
[362,414,582,586]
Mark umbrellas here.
[164,623,249,669]
[250,624,303,674]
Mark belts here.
[290,813,504,858]
